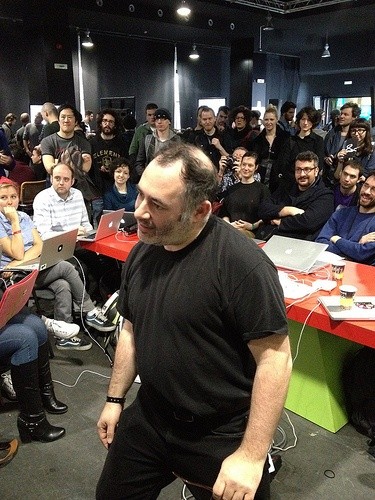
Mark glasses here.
[155,108,172,121]
[156,118,168,121]
[351,129,367,134]
[102,120,114,125]
[296,166,317,173]
[236,116,245,119]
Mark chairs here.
[18,179,48,215]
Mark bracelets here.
[252,224,254,230]
[12,230,21,235]
[106,396,126,403]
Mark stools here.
[28,288,56,317]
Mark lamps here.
[176,1,191,15]
[188,43,199,59]
[81,30,94,47]
[321,30,330,57]
[263,13,274,30]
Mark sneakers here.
[45,317,79,339]
[85,311,116,331]
[56,336,94,351]
[0,438,18,468]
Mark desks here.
[80,221,375,433]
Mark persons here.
[0,279,80,400]
[0,439,18,467]
[0,314,68,443]
[95,143,293,500]
[0,177,116,350]
[33,163,110,302]
[0,101,375,267]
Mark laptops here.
[78,208,137,242]
[261,235,341,273]
[10,227,78,271]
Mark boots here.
[10,358,65,442]
[37,340,69,414]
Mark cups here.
[331,261,347,280]
[338,285,357,310]
